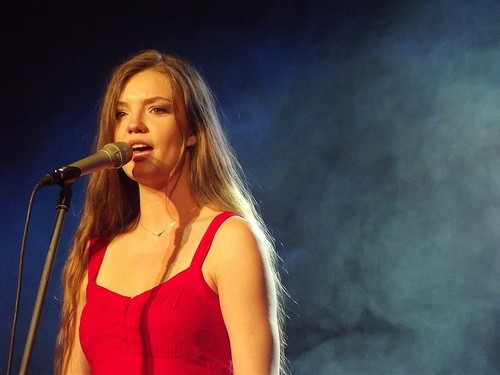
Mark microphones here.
[38,141,133,188]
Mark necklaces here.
[137,203,201,238]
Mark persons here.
[51,48,302,375]
